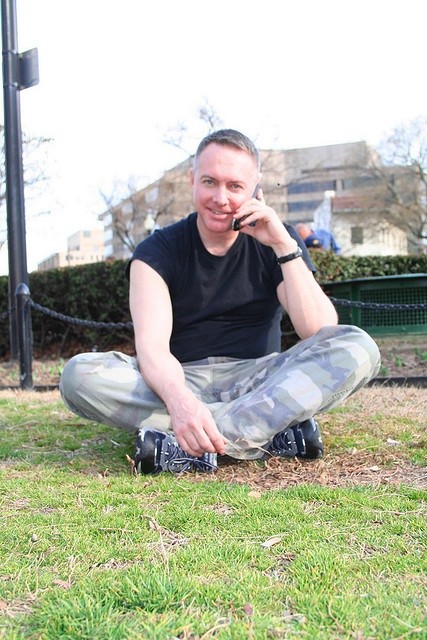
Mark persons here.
[296,222,341,255]
[59,130,382,477]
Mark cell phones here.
[231,183,260,230]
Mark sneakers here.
[265,417,324,462]
[133,425,219,478]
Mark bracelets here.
[277,247,304,262]
